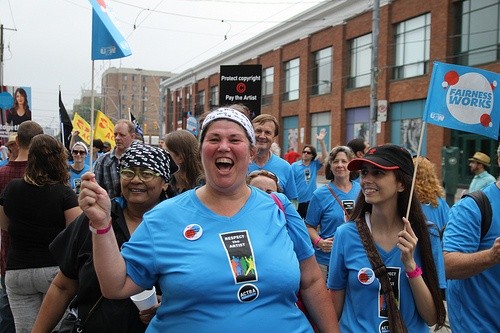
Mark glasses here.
[120,168,161,182]
[73,150,86,155]
[303,151,312,154]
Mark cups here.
[130,285,158,312]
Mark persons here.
[6,88,32,125]
[30,145,180,333]
[0,113,452,303]
[327,145,447,333]
[442,145,500,333]
[0,135,84,333]
[79,104,339,333]
[468,152,497,193]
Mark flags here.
[89,0,132,61]
[93,110,116,148]
[430,63,500,141]
[130,111,144,143]
[71,112,94,145]
[59,90,73,149]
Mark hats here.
[103,142,111,148]
[468,152,491,166]
[347,143,414,177]
[119,142,173,183]
